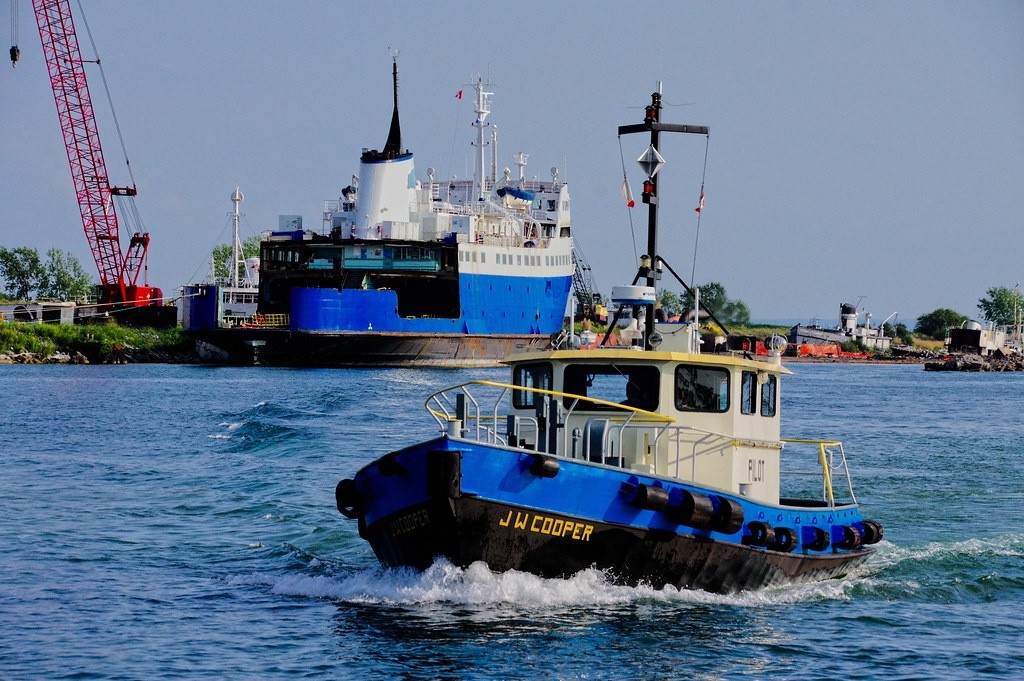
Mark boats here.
[182,180,261,332]
[787,296,898,356]
[190,41,577,369]
[335,77,885,594]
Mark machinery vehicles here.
[10,0,163,313]
